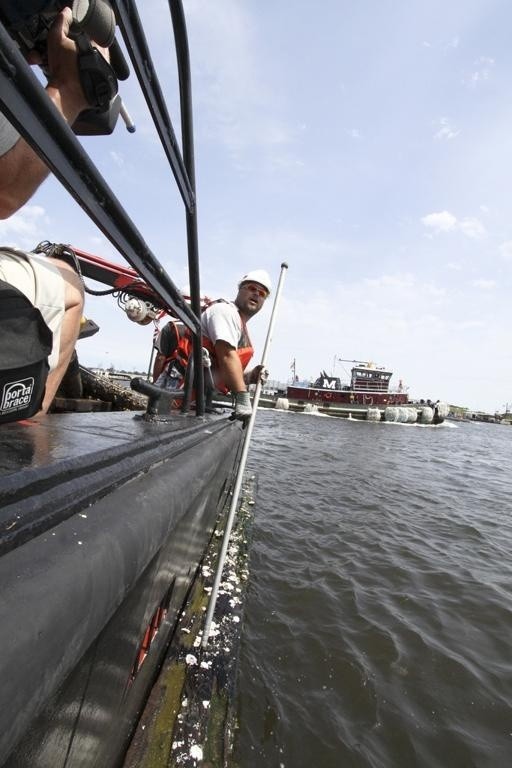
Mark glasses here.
[241,284,266,297]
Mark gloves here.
[244,365,270,386]
[228,393,253,429]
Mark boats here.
[214,356,450,425]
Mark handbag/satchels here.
[1,280,53,423]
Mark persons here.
[0,0,110,420]
[153,270,270,429]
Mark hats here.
[238,269,270,292]
